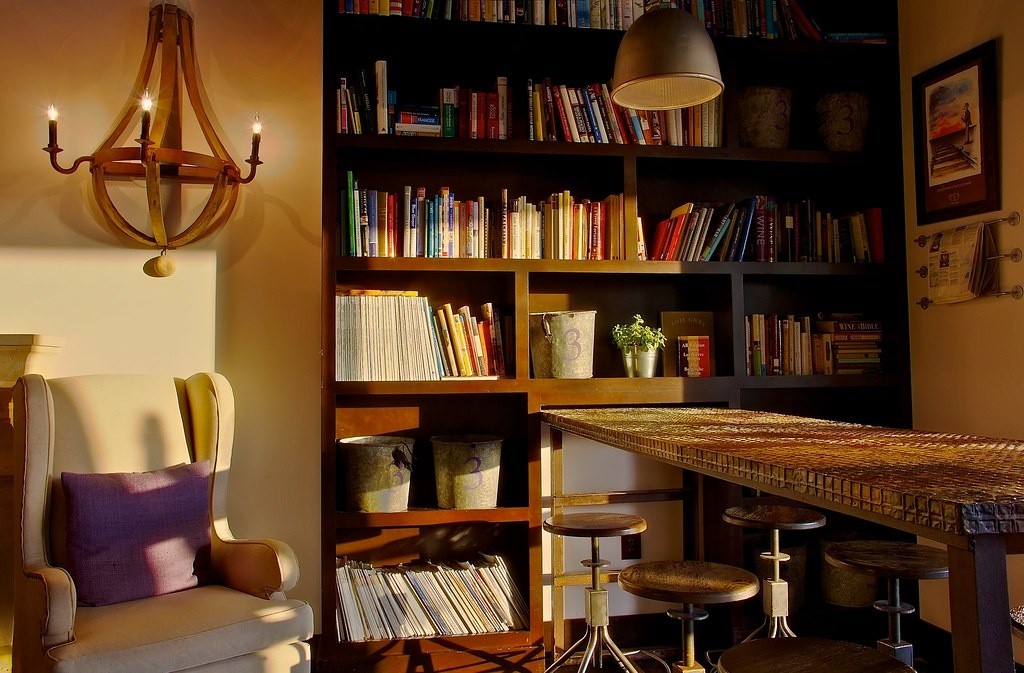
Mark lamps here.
[609,0,722,111]
[42,0,264,277]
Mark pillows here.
[58,459,211,606]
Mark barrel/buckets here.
[737,86,791,149]
[338,435,415,514]
[821,537,881,609]
[817,91,870,152]
[431,431,505,510]
[752,541,806,612]
[529,310,594,380]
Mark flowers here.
[611,313,666,349]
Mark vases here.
[621,346,659,379]
[817,93,866,150]
[737,87,790,148]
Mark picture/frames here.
[911,36,1002,227]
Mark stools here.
[619,561,759,673]
[825,542,949,665]
[724,506,826,641]
[1010,604,1024,673]
[718,638,917,673]
[542,513,648,673]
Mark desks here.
[541,406,1024,673]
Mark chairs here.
[6,373,314,673]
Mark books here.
[335,551,530,642]
[339,0,889,44]
[337,61,719,148]
[677,336,710,377]
[335,289,506,381]
[661,311,716,377]
[348,170,624,261]
[637,195,884,262]
[745,313,883,376]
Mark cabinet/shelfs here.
[321,0,910,673]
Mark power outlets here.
[621,533,642,559]
